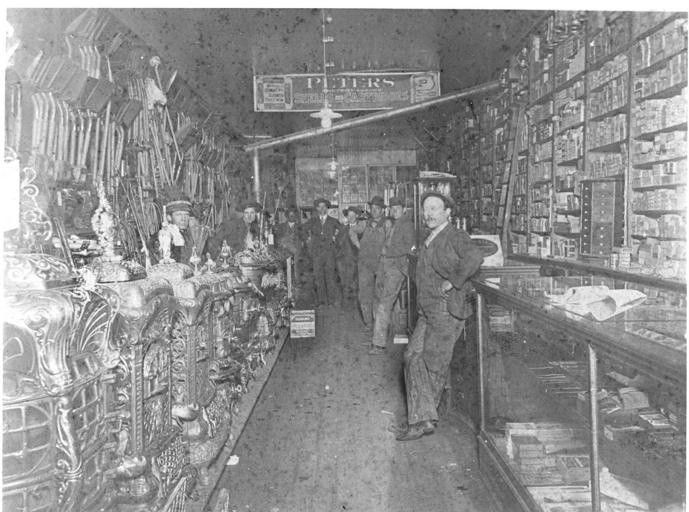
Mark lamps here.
[326,132,339,171]
[310,8,342,129]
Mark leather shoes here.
[361,338,389,355]
[388,417,440,441]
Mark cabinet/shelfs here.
[408,10,686,512]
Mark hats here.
[165,190,457,218]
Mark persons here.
[395,191,484,440]
[217,196,417,356]
[147,199,195,263]
